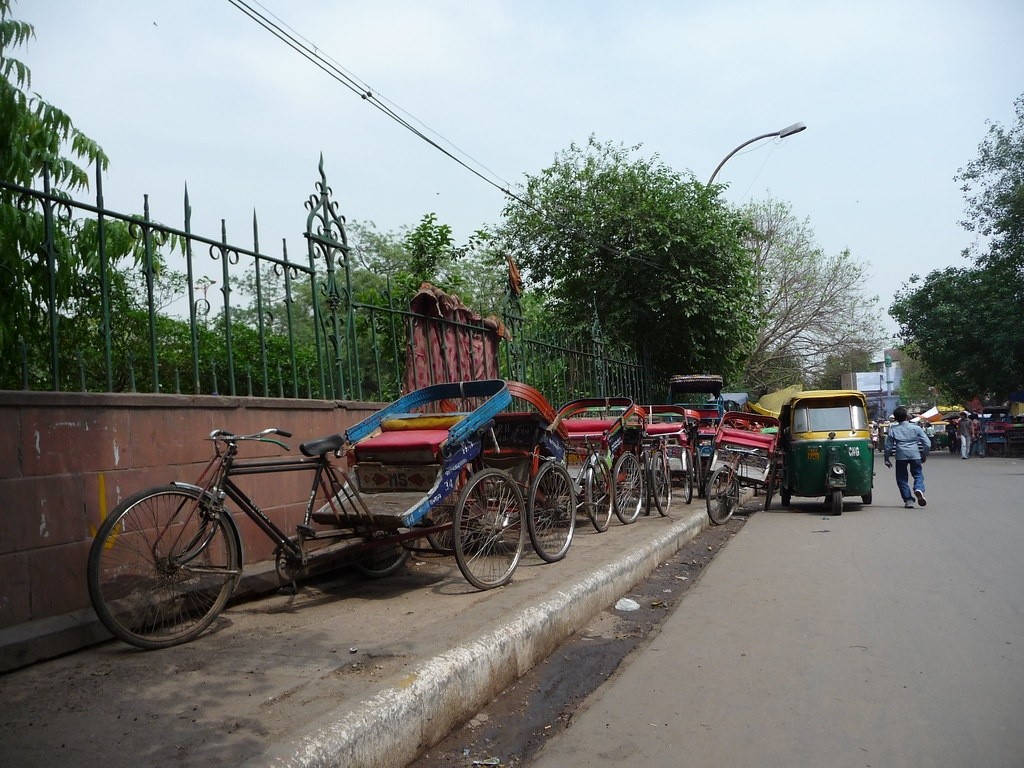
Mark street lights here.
[706,121,806,187]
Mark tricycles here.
[85,378,528,650]
[703,410,782,525]
[556,375,750,525]
[422,380,615,564]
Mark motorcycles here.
[776,390,876,517]
[868,421,950,453]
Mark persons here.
[967,414,983,457]
[868,414,935,453]
[954,412,974,459]
[884,406,930,508]
[945,419,958,454]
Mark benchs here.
[717,428,775,459]
[560,420,615,466]
[355,428,449,491]
[645,421,686,444]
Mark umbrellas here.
[940,412,960,419]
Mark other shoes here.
[915,489,927,506]
[980,453,982,455]
[905,500,913,507]
[962,456,968,458]
